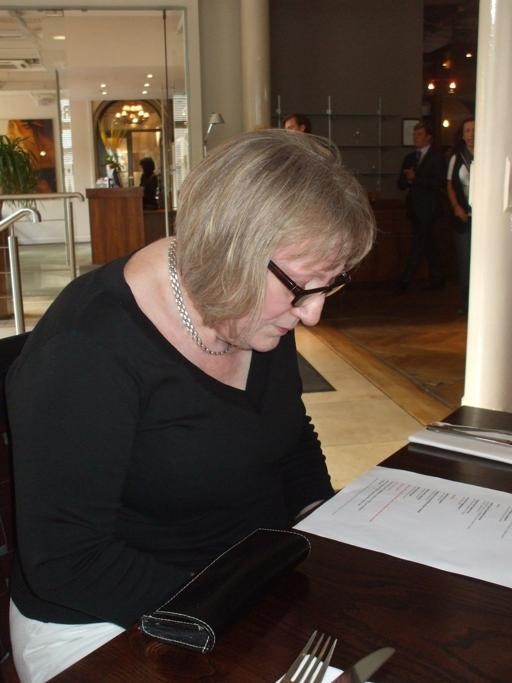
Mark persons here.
[445,118,477,313]
[136,154,160,212]
[396,120,447,284]
[4,128,379,681]
[282,112,314,136]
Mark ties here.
[413,151,421,172]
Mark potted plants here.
[0,132,45,320]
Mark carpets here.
[295,345,337,393]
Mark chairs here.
[0,332,33,680]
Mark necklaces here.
[167,238,233,356]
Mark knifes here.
[333,643,397,681]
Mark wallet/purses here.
[140,527,312,655]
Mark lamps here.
[448,78,458,95]
[202,111,226,158]
[114,99,150,124]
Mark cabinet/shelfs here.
[273,93,402,188]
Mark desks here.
[44,404,510,678]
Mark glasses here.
[267,259,352,308]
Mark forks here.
[280,628,338,683]
